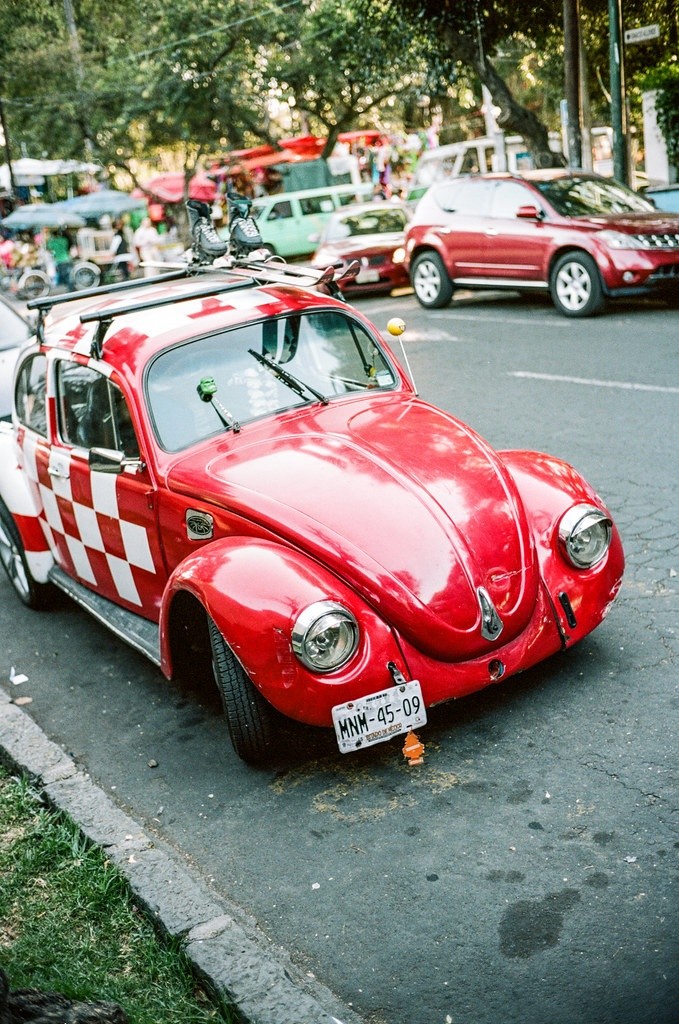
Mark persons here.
[253,172,271,214]
[0,215,181,289]
[356,135,399,196]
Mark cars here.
[404,127,614,212]
[307,199,413,298]
[0,271,625,764]
[0,293,38,428]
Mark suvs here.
[402,169,679,319]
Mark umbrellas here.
[0,158,102,188]
[2,174,227,230]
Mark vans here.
[250,182,374,264]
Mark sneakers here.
[225,192,263,247]
[185,199,228,258]
